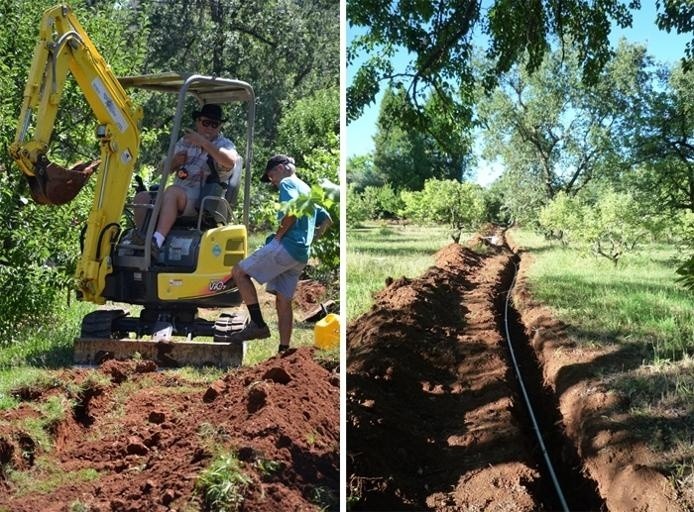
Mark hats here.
[192,104,229,122]
[260,155,295,182]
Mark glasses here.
[198,118,222,128]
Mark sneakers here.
[132,230,160,258]
[231,321,271,339]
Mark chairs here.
[148,157,245,230]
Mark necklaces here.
[228,153,333,352]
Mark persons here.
[129,102,239,260]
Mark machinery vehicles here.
[6,3,257,370]
